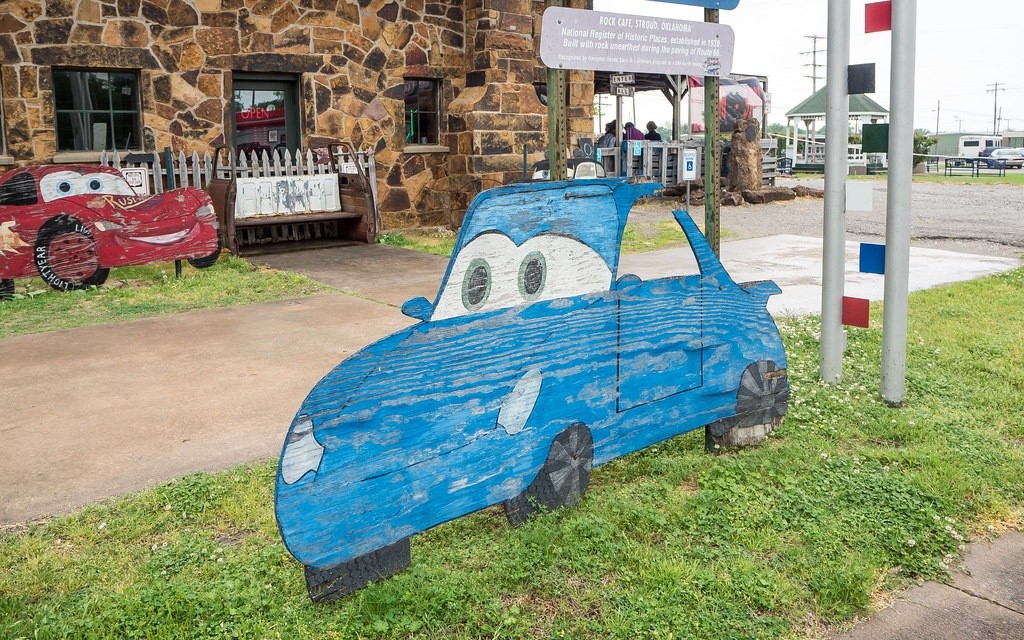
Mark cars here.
[981,147,1002,164]
[987,148,1024,169]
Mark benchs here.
[235,210,362,251]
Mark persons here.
[597,120,661,171]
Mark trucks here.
[958,135,1003,158]
